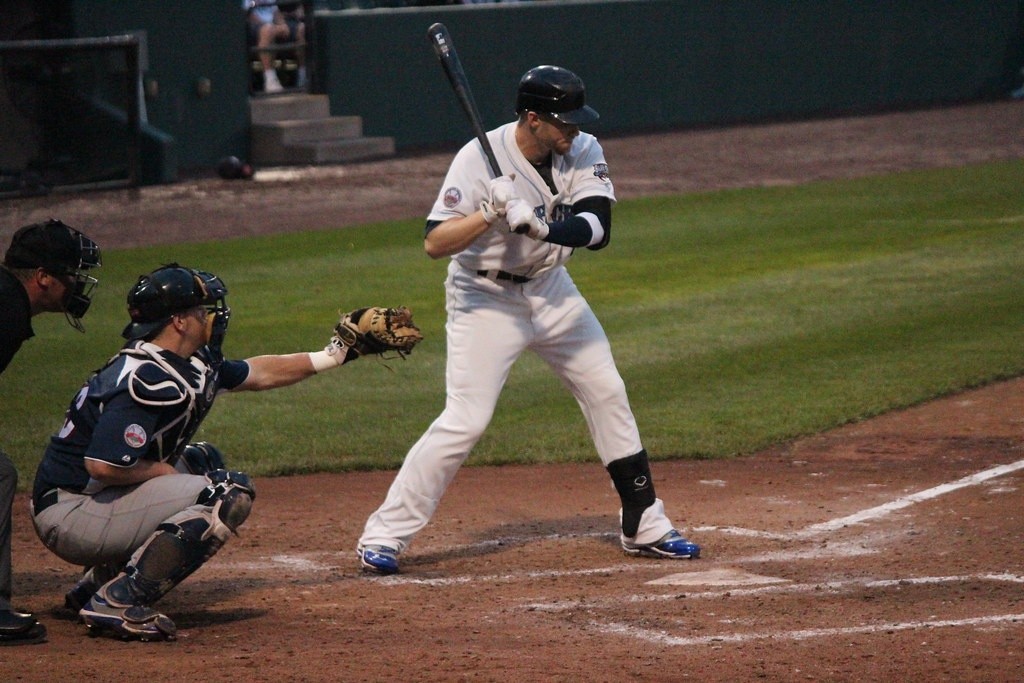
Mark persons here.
[0,218,103,646]
[245,0,306,94]
[356,63,701,576]
[27,262,425,642]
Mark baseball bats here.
[426,21,533,237]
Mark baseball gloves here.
[326,301,425,363]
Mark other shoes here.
[294,73,311,88]
[263,77,285,94]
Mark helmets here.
[5,217,104,269]
[119,262,204,339]
[513,64,601,125]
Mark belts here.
[476,269,533,284]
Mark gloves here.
[501,199,551,242]
[480,173,517,225]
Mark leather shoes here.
[0,608,49,644]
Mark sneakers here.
[78,580,177,640]
[64,577,103,610]
[356,541,399,575]
[620,523,701,559]
